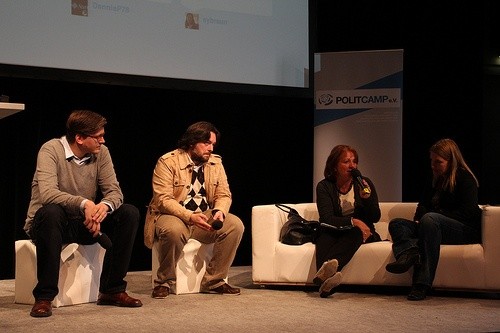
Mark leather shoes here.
[30,298,52,317]
[97,290,143,307]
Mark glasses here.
[79,130,104,139]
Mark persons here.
[23,110,143,317]
[144,121,245,299]
[185,13,198,29]
[386,138,479,301]
[313,144,381,298]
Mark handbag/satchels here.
[275,204,318,245]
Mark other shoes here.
[313,259,342,297]
[200,281,240,295]
[153,286,169,299]
[386,253,427,274]
[407,286,426,300]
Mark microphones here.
[208,216,223,230]
[76,206,113,250]
[351,167,371,194]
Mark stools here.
[14,240,106,307]
[152,238,228,294]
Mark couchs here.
[252,201,500,292]
